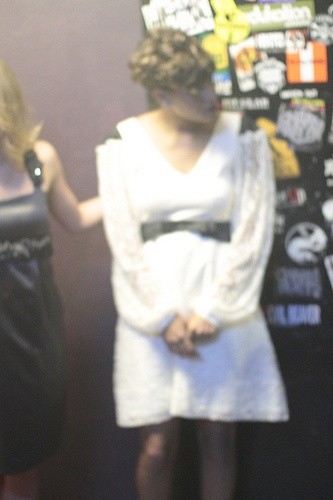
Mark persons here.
[0,59,103,500]
[95,28,290,500]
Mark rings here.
[179,336,185,345]
